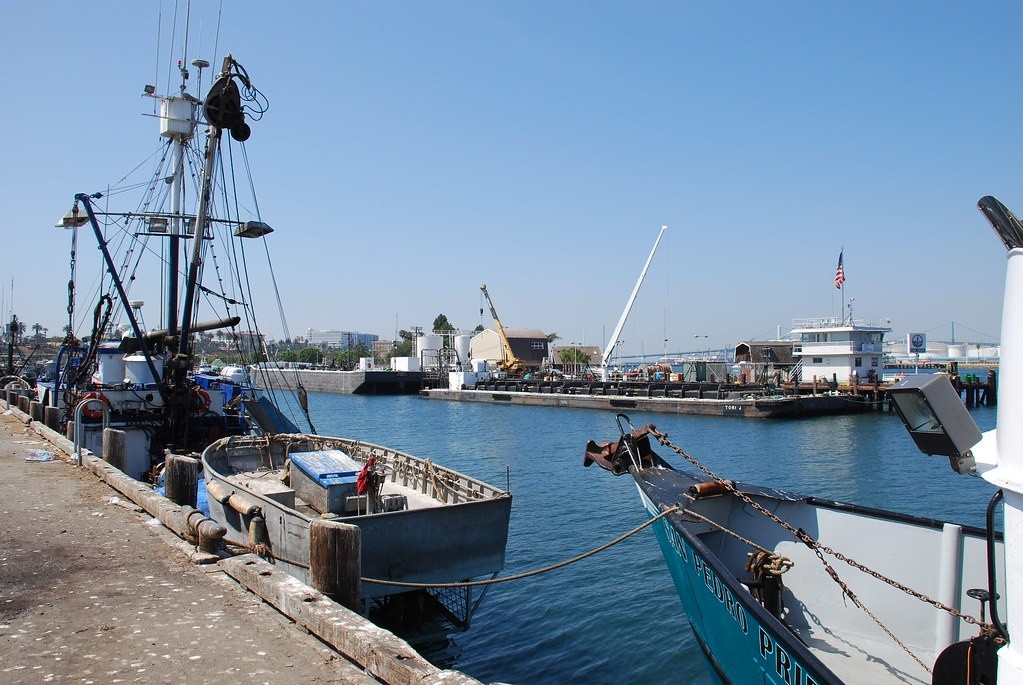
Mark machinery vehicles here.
[587,224,668,395]
[477,285,530,378]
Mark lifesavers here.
[191,388,211,412]
[81,392,110,419]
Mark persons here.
[588,373,596,381]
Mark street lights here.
[694,335,709,356]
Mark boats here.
[248,354,430,394]
[200,431,511,635]
[581,196,1023,685]
[37,1,255,483]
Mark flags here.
[835,252,845,289]
